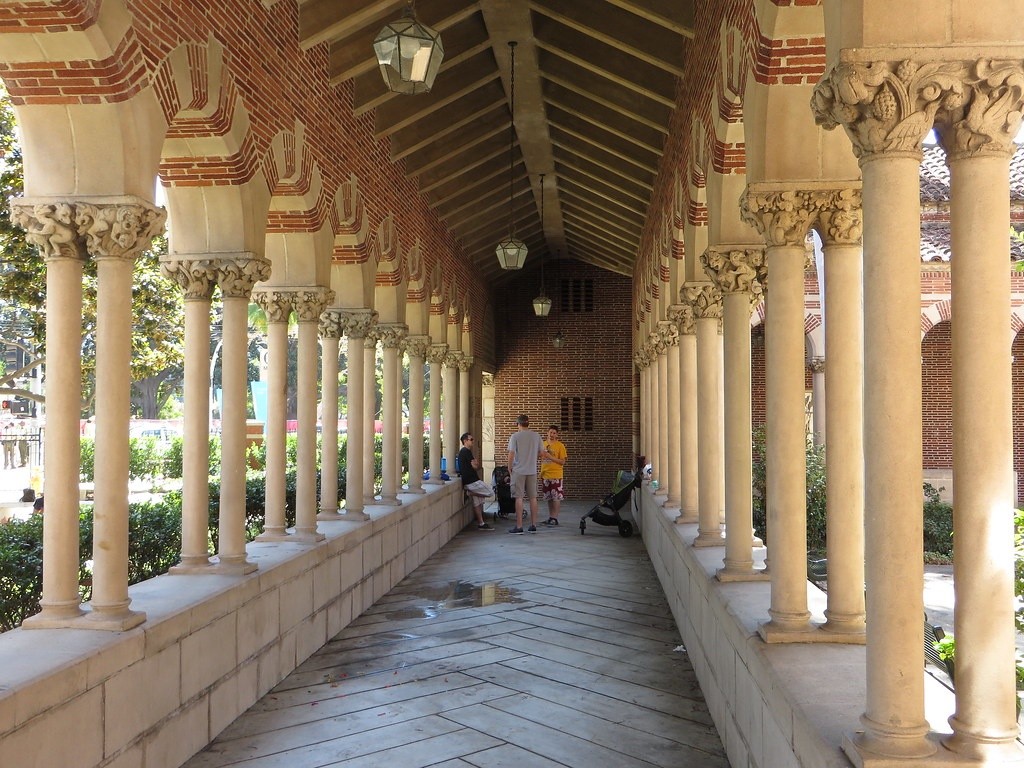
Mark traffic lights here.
[2,400,11,409]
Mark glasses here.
[516,422,519,426]
[466,438,474,441]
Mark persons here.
[458,432,494,531]
[507,414,544,534]
[2,420,29,469]
[539,425,568,527]
[32,496,44,515]
[84,420,91,436]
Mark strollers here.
[579,466,643,538]
[491,465,528,522]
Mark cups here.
[652,480,658,488]
[544,450,549,453]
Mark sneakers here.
[527,526,537,534]
[463,489,469,506]
[508,526,525,535]
[477,523,494,531]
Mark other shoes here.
[541,517,559,527]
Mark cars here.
[128,421,182,454]
